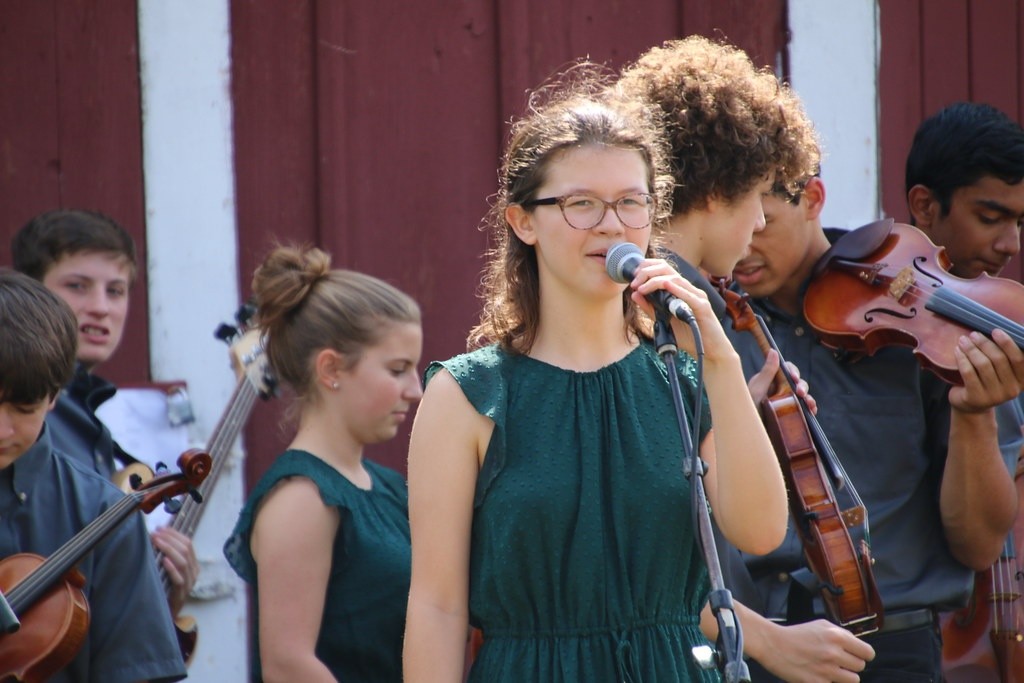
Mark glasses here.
[521,193,658,232]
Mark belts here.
[873,604,935,638]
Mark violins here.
[704,268,887,640]
[932,429,1024,682]
[800,216,1024,388]
[1,446,214,683]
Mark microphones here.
[605,242,695,324]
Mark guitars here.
[153,303,284,601]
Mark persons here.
[9,210,201,618]
[904,101,1024,477]
[223,247,422,683]
[403,96,788,683]
[719,164,1019,683]
[0,267,189,683]
[612,35,876,683]
